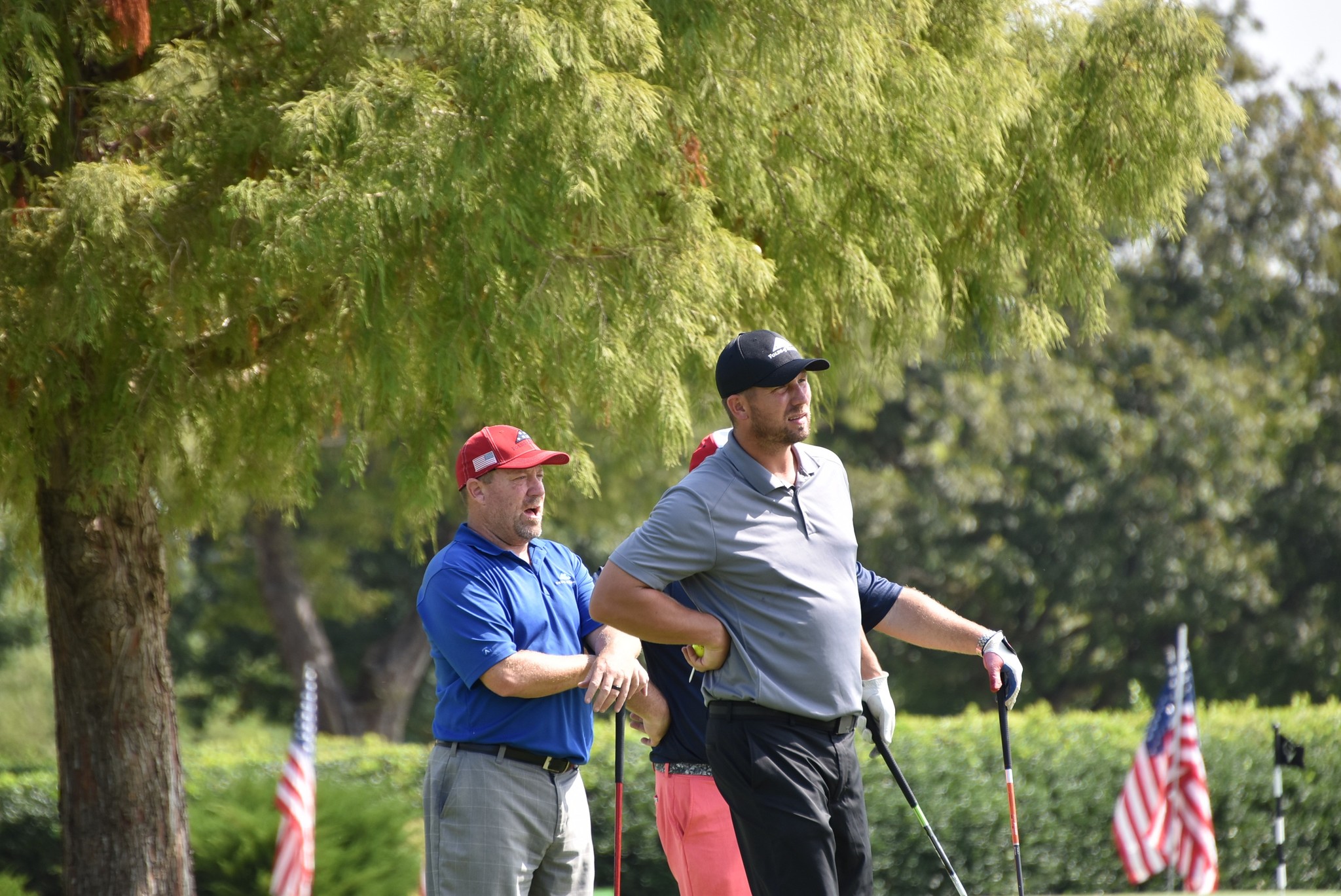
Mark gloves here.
[983,629,1023,712]
[856,671,896,759]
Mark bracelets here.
[975,629,999,656]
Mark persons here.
[584,329,896,896]
[415,424,648,896]
[625,427,1025,896]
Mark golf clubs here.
[995,669,1025,896]
[861,700,968,896]
[613,697,627,895]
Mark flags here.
[1112,645,1220,896]
[270,663,319,896]
[1273,733,1305,772]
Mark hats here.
[457,424,570,493]
[688,426,735,475]
[715,330,831,399]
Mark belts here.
[654,761,713,777]
[708,701,859,736]
[434,739,579,774]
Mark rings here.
[612,684,622,691]
